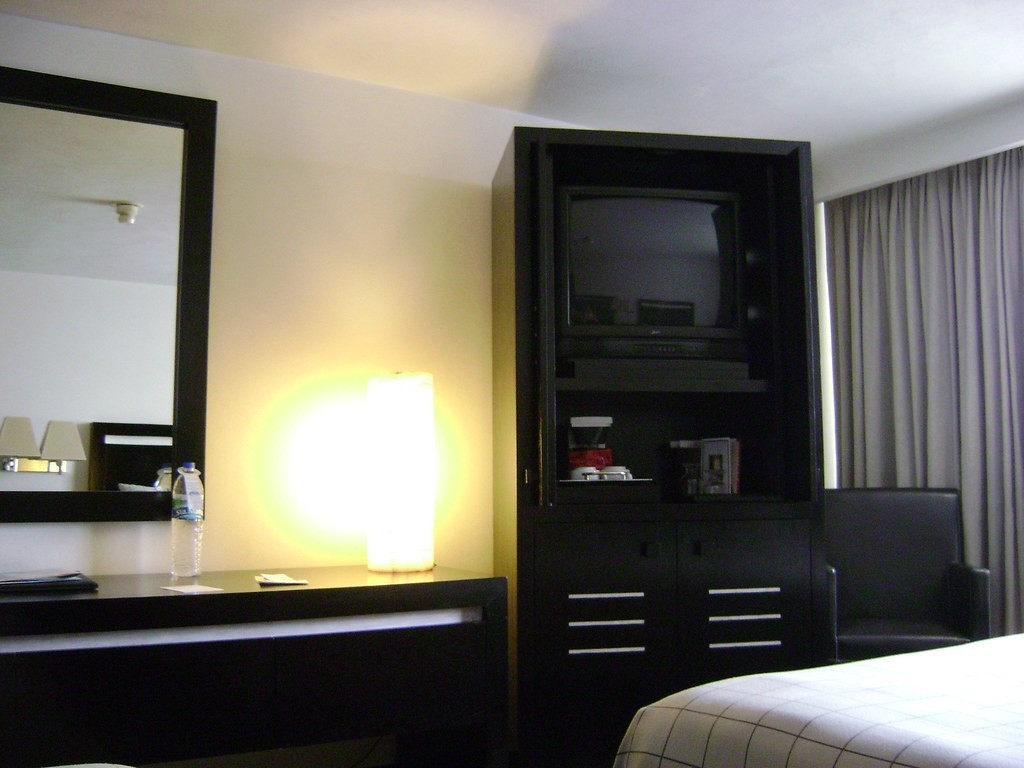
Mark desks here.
[0,567,509,768]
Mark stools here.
[139,722,396,768]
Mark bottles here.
[170,462,205,577]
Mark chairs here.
[88,420,173,491]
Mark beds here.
[614,631,1024,768]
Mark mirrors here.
[0,66,217,522]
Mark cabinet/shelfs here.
[1,471,87,492]
[491,123,821,768]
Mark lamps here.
[41,421,85,474]
[0,417,40,471]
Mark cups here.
[572,466,601,480]
[602,465,632,480]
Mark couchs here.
[822,488,992,666]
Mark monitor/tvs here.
[558,186,748,358]
